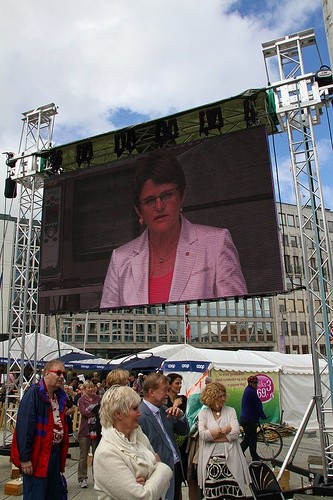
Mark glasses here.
[47,369,67,377]
[252,381,258,383]
[138,185,182,206]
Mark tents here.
[163,347,333,429]
[0,332,195,372]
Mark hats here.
[247,375,259,382]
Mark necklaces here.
[150,241,177,263]
[214,410,221,415]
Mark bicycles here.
[237,415,284,461]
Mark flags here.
[185,304,191,340]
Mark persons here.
[138,373,190,500]
[166,373,188,486]
[240,376,270,467]
[77,379,102,488]
[93,385,173,500]
[197,382,255,500]
[10,359,69,500]
[0,369,18,431]
[106,368,129,388]
[65,372,147,437]
[100,154,248,308]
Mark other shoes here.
[79,480,88,489]
